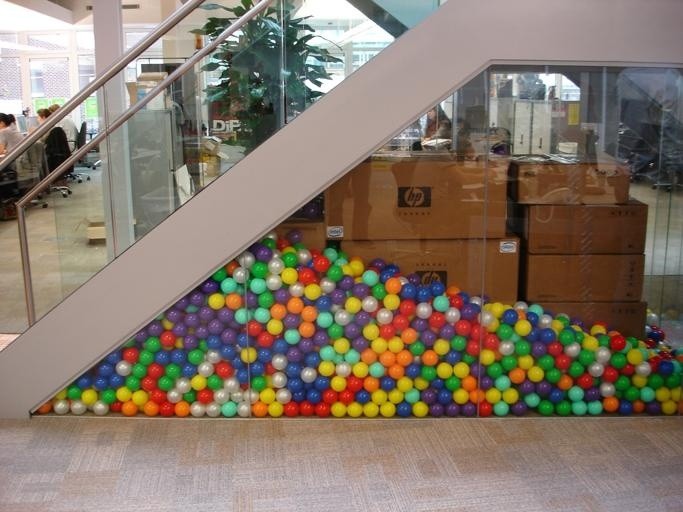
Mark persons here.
[8,114,17,130]
[425,104,451,140]
[28,108,74,173]
[48,104,79,152]
[0,112,27,170]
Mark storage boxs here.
[128,73,167,111]
[339,235,520,310]
[519,254,646,302]
[322,155,508,240]
[278,218,326,254]
[508,156,632,205]
[507,198,648,255]
[537,302,648,339]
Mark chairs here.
[1,123,95,208]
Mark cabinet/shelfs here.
[498,99,553,155]
